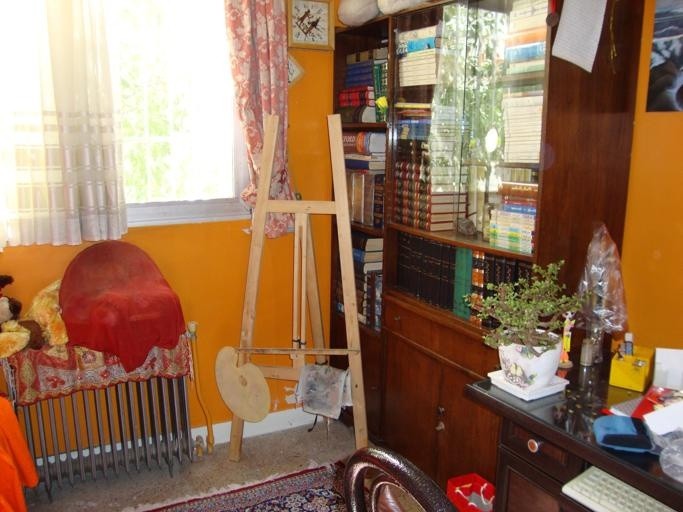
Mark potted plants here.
[461,259,592,393]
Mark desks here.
[459,345,682,512]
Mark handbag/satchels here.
[445,472,494,512]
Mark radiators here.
[0,323,205,492]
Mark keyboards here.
[559,465,677,512]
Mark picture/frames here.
[284,0,333,51]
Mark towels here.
[294,361,355,422]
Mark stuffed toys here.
[0,275,44,362]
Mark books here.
[331,1,552,335]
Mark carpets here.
[113,463,346,511]
